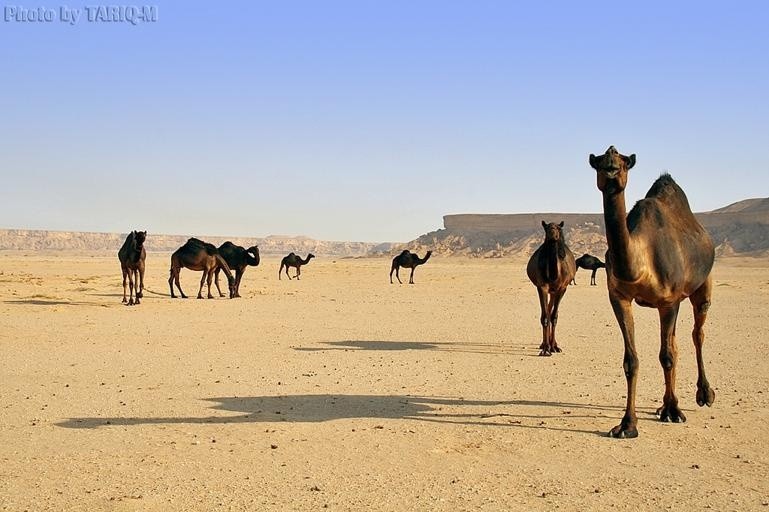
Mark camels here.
[278,251,315,281]
[167,237,261,300]
[569,253,607,287]
[117,229,148,306]
[587,141,718,439]
[524,219,579,358]
[388,249,434,285]
